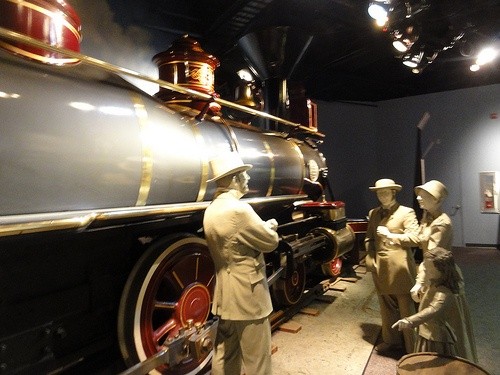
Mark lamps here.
[368,0,476,74]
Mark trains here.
[0,0,357,375]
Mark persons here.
[392,248,461,356]
[364,179,419,354]
[203,152,279,375]
[409,180,479,363]
